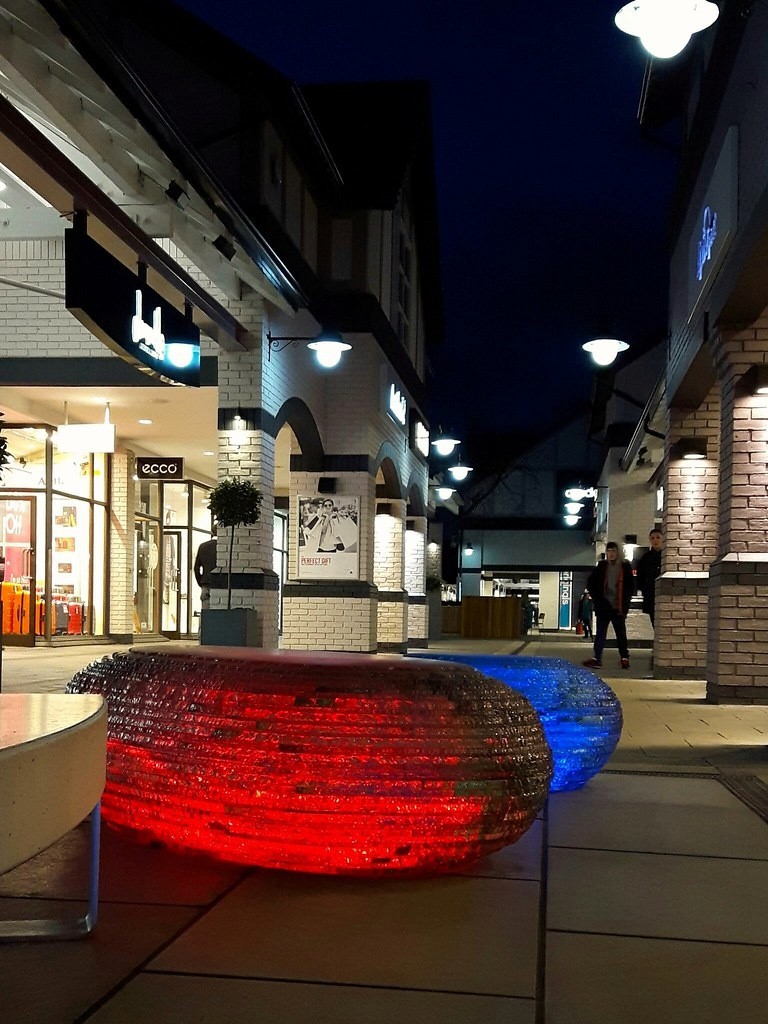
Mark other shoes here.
[590,634,592,638]
[583,636,588,638]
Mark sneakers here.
[621,656,630,670]
[582,657,602,669]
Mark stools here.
[0,693,109,941]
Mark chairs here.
[531,613,545,635]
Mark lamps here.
[464,543,474,555]
[564,501,602,514]
[317,477,337,493]
[406,520,415,530]
[429,453,473,480]
[681,437,707,459]
[625,535,636,544]
[428,479,457,499]
[430,422,461,457]
[565,480,609,500]
[234,407,242,421]
[614,0,755,58]
[564,514,595,525]
[164,322,199,367]
[750,363,768,394]
[265,324,352,368]
[377,503,391,516]
[581,316,672,366]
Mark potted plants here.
[201,475,264,646]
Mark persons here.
[305,499,343,552]
[576,593,593,642]
[194,525,218,643]
[637,529,662,628]
[582,539,633,669]
